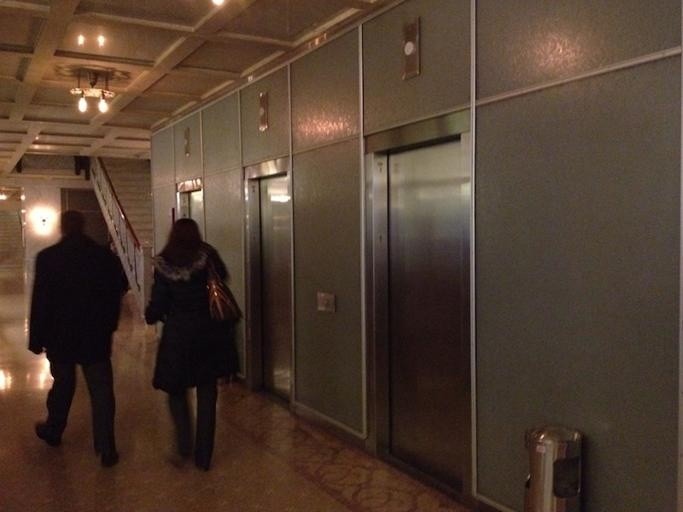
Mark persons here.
[145,218,241,469]
[27,207,129,470]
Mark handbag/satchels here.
[200,259,244,323]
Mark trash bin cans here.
[524,423,584,512]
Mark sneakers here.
[34,421,61,448]
[168,443,210,472]
[101,447,119,466]
